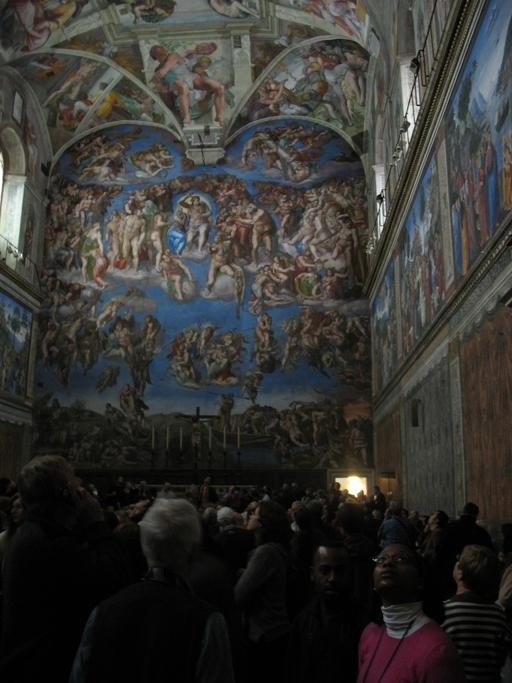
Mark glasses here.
[374,555,409,565]
[455,554,460,561]
[315,563,343,577]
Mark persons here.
[1,458,510,682]
[0,0,369,471]
